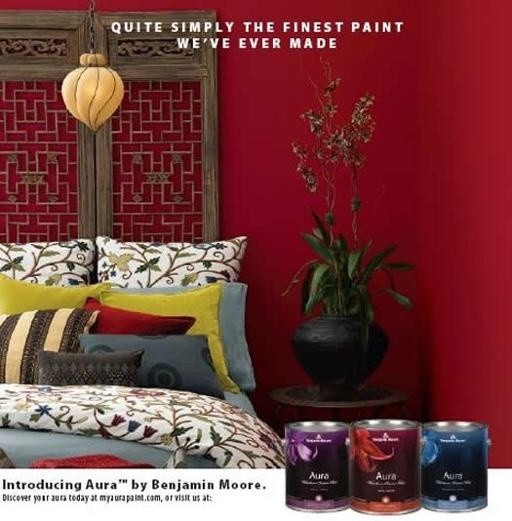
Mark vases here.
[290,316,390,396]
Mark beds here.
[1,391,286,470]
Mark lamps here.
[60,1,125,137]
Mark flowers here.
[278,51,418,352]
